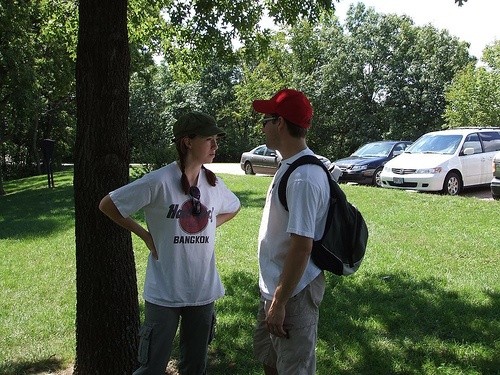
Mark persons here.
[252,89,332,375]
[99,112,241,375]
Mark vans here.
[379,126,500,196]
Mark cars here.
[240,144,331,176]
[490,151,500,201]
[328,140,415,187]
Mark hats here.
[253,89,312,128]
[173,111,226,138]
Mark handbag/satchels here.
[277,155,369,276]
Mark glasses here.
[263,116,277,126]
[189,187,202,216]
